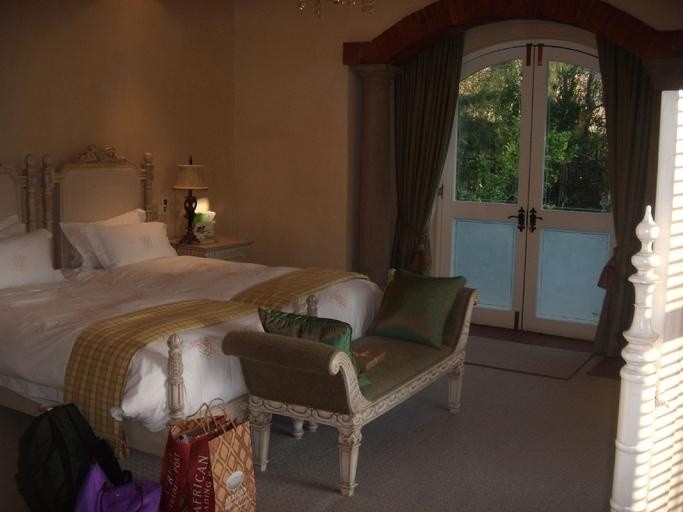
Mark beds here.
[0,144,398,477]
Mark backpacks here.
[14,402,134,512]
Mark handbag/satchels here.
[160,399,232,512]
[189,404,256,512]
[71,456,163,512]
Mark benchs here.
[221,268,478,497]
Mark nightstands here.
[175,238,254,264]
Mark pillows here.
[83,222,179,269]
[366,267,468,350]
[0,228,57,293]
[257,307,388,389]
[0,214,18,226]
[59,208,147,269]
[0,223,26,235]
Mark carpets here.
[464,323,594,380]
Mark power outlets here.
[161,198,168,207]
[160,207,169,216]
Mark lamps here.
[172,154,209,245]
